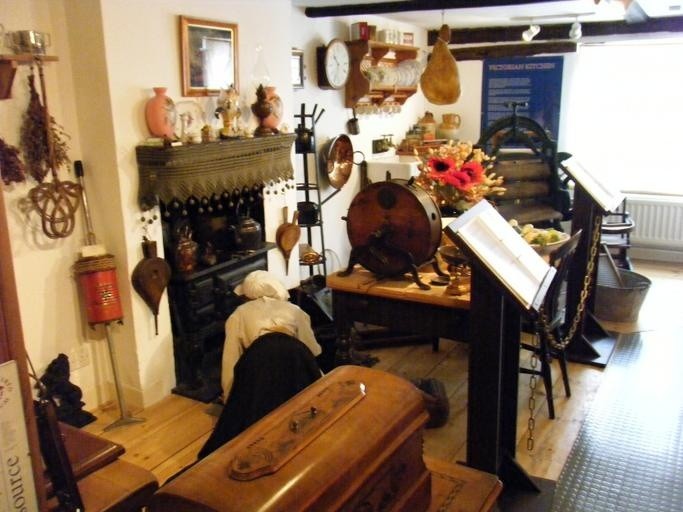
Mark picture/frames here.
[294,49,303,88]
[179,15,240,97]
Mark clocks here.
[316,38,350,90]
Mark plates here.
[522,226,572,257]
[319,134,354,190]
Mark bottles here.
[417,111,436,139]
[436,113,462,141]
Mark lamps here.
[512,10,596,43]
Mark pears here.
[537,232,550,245]
[548,228,560,243]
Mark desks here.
[420,455,504,512]
[326,251,562,368]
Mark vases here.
[440,206,462,217]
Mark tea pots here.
[228,200,263,253]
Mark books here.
[461,210,550,307]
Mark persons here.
[198,269,324,456]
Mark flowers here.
[413,140,506,213]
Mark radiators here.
[602,199,682,248]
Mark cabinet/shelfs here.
[346,38,420,109]
[165,241,276,404]
[294,104,326,278]
[0,178,157,512]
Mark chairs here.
[519,228,583,419]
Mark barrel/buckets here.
[593,253,652,322]
[490,151,551,206]
